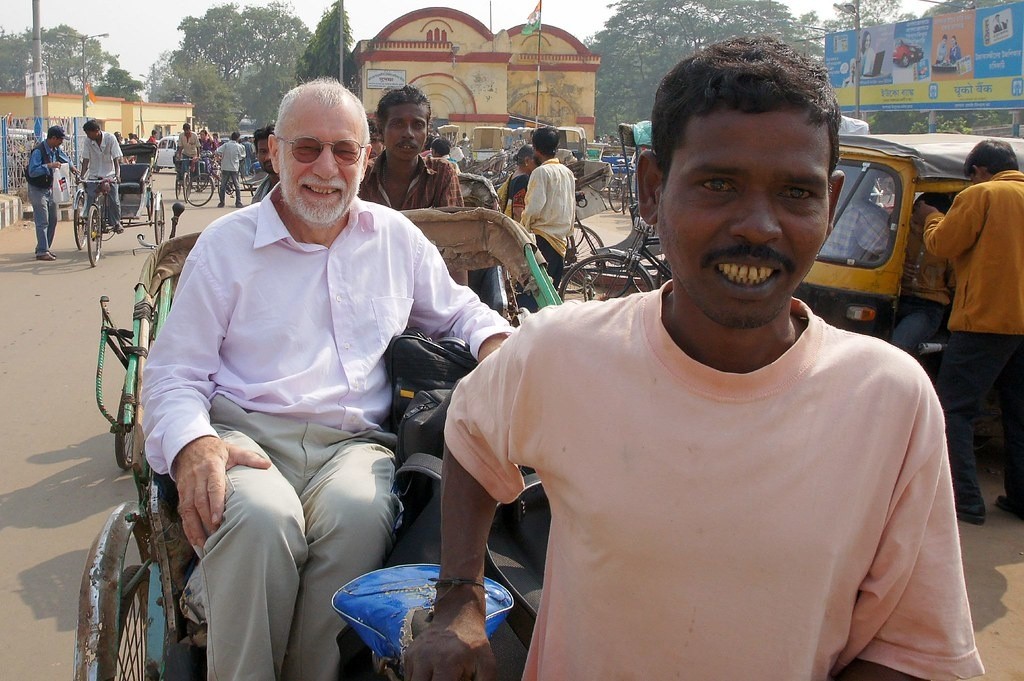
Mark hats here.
[47,126,72,141]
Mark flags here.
[522,1,541,34]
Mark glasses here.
[278,132,366,165]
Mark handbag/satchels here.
[52,163,70,204]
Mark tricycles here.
[172,139,268,207]
[75,205,563,681]
[73,142,166,269]
[558,117,672,302]
[436,123,642,295]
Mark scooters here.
[791,132,1024,453]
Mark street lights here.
[55,32,110,116]
[832,2,861,120]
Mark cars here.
[893,38,924,67]
[155,136,179,172]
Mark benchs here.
[132,209,583,648]
[87,160,152,197]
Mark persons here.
[172,123,469,209]
[113,129,158,173]
[358,85,468,287]
[595,133,617,146]
[818,166,956,357]
[403,35,995,681]
[25,125,81,261]
[79,120,124,234]
[140,77,519,681]
[503,125,577,313]
[909,138,1024,524]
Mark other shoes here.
[115,222,124,234]
[217,202,225,208]
[955,493,1023,526]
[35,247,57,261]
[235,201,243,208]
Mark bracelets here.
[427,577,485,606]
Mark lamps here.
[450,44,460,71]
